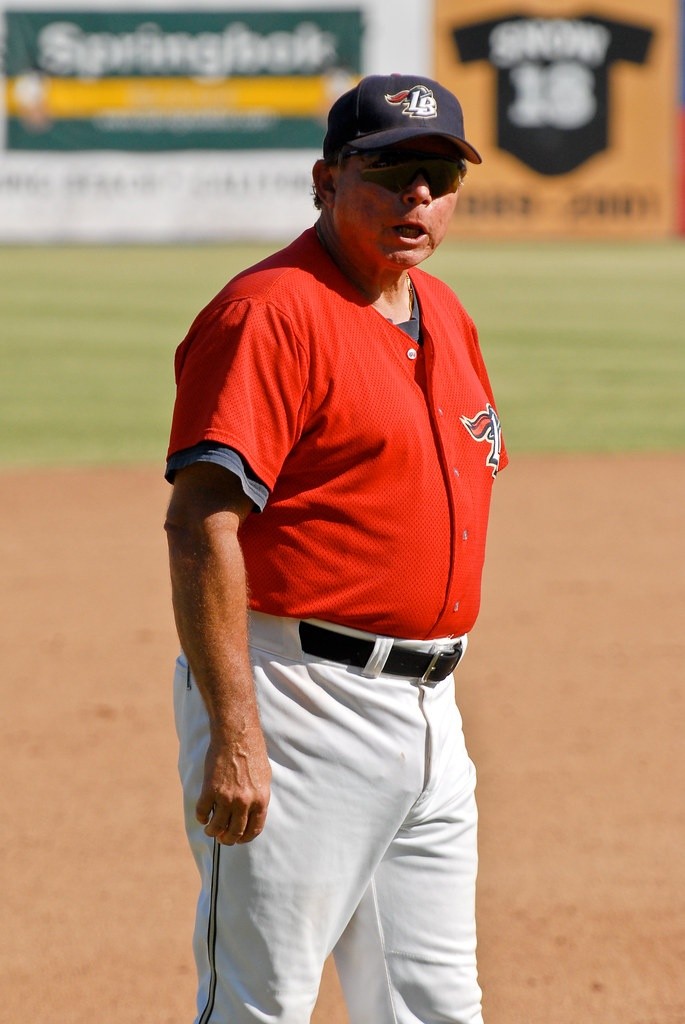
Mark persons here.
[165,74,507,1024]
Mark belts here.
[298,621,461,685]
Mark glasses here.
[344,145,466,196]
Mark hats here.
[323,74,482,165]
